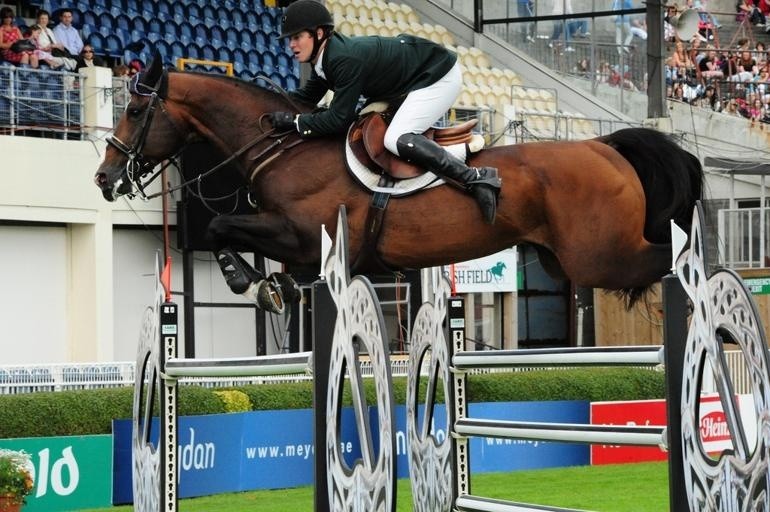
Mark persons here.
[516,0,535,43]
[271,1,499,227]
[1,6,145,105]
[574,0,770,123]
[560,14,590,38]
[547,0,575,52]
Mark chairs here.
[3,1,302,139]
[320,1,599,144]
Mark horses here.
[93,48,738,345]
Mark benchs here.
[596,1,770,95]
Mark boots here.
[396,132,502,226]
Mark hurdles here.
[131,278,397,512]
[406,270,770,512]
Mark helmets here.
[274,0,335,40]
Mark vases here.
[0,492,33,512]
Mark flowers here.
[0,451,34,492]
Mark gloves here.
[263,110,298,132]
